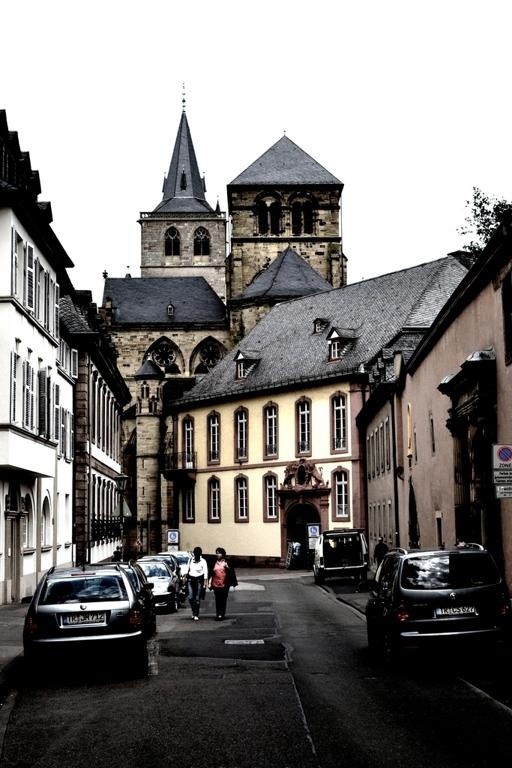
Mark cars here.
[365,538,508,662]
[21,548,199,681]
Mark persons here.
[185,547,208,621]
[208,548,238,620]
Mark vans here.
[313,527,371,586]
